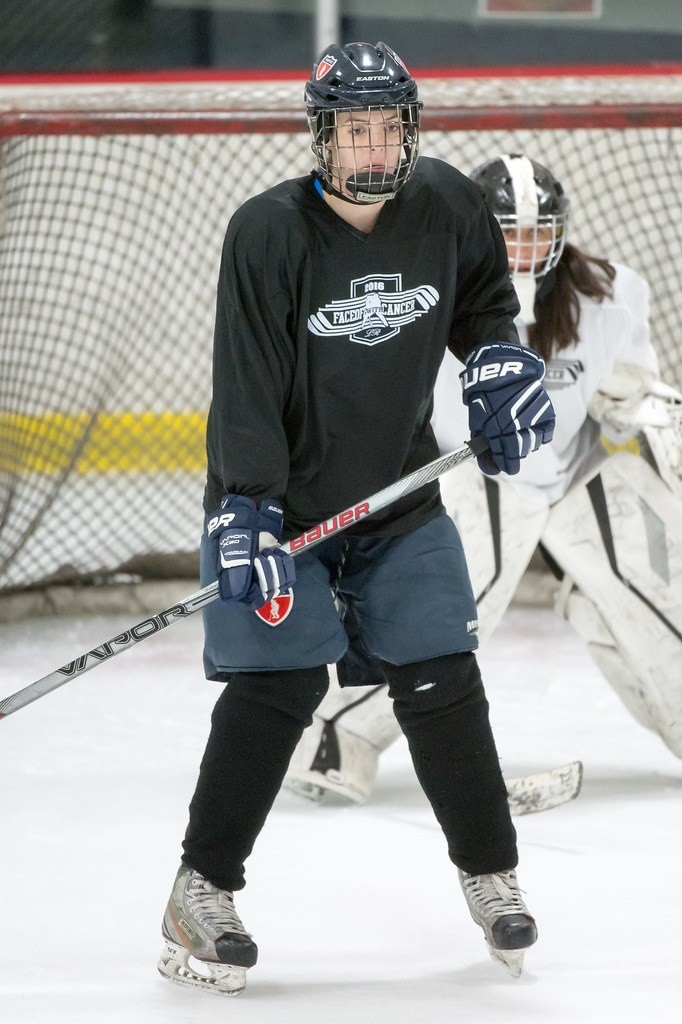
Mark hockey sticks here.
[504,761,583,818]
[0,436,476,724]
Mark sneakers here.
[459,859,536,979]
[155,863,256,997]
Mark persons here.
[158,34,542,992]
[282,152,682,816]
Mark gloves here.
[462,343,554,474]
[207,495,295,609]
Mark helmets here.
[476,153,565,274]
[303,41,424,203]
